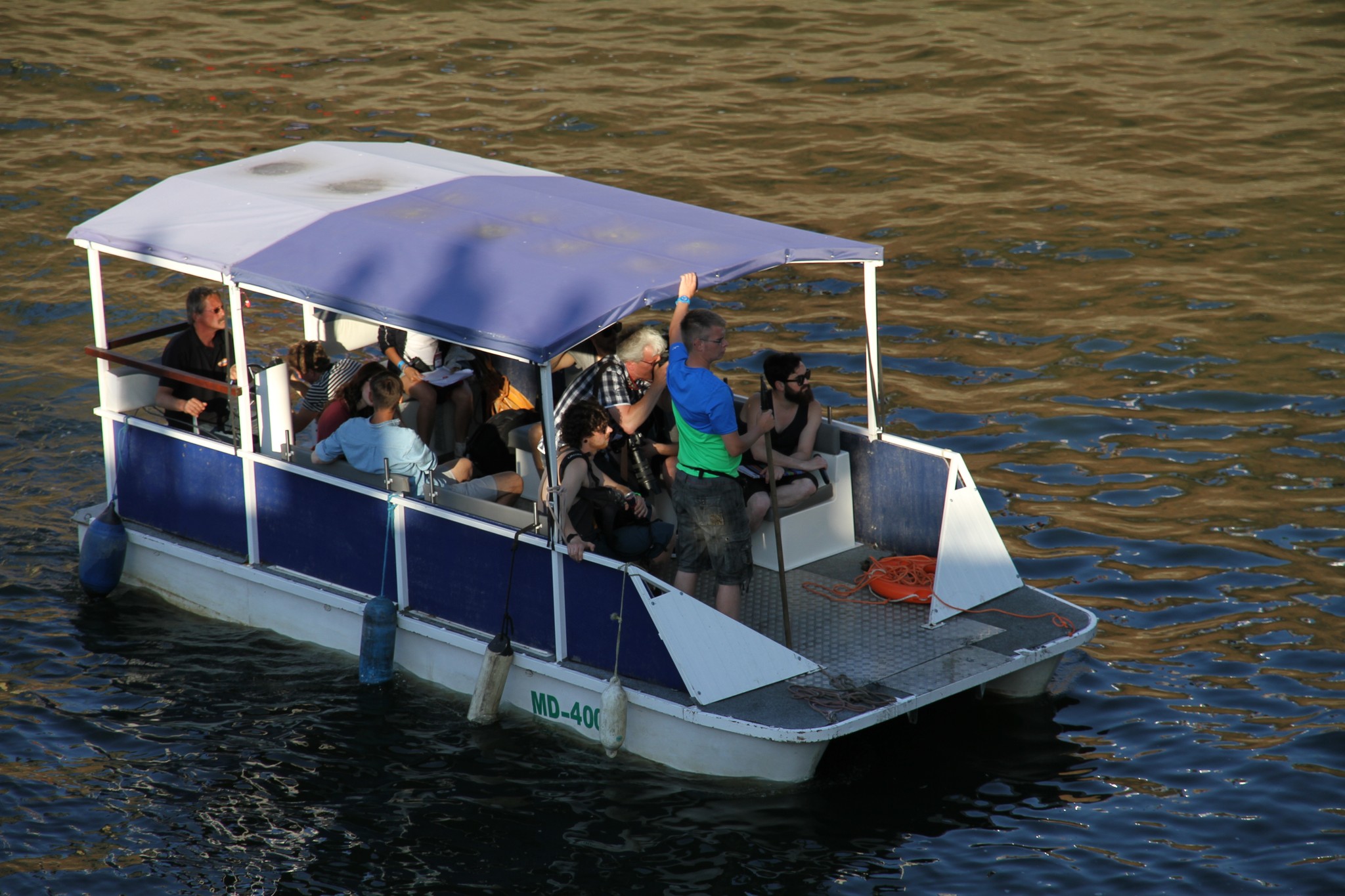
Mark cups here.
[624,491,637,510]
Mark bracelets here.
[398,360,405,370]
[402,364,408,374]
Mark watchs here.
[566,534,579,543]
[676,296,691,305]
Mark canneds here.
[624,492,638,511]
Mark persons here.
[289,271,829,628]
[155,287,237,440]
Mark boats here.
[69,141,1097,787]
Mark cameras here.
[612,431,660,496]
[651,351,669,381]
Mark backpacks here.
[560,452,626,540]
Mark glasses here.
[785,369,811,386]
[694,337,725,346]
[640,360,657,367]
[594,424,608,435]
[203,305,224,314]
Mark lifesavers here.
[868,556,934,603]
